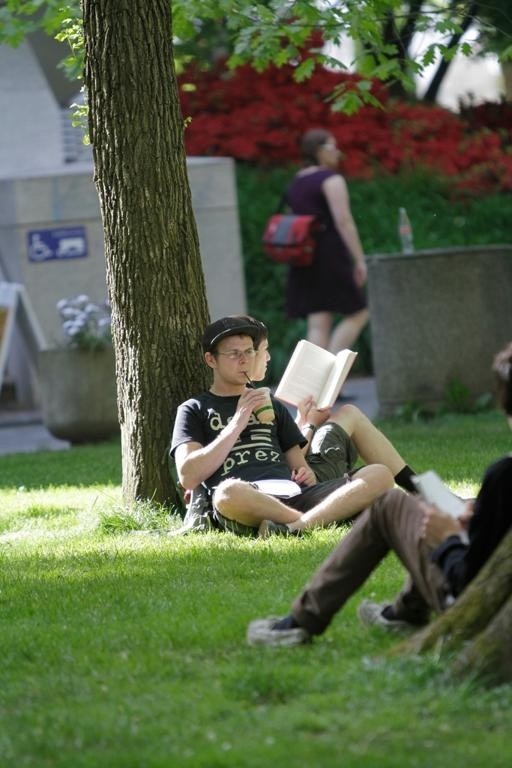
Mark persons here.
[166,315,395,537]
[235,315,419,497]
[285,127,369,403]
[244,342,512,650]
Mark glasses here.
[209,349,258,359]
[321,145,339,151]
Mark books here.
[273,339,359,412]
[411,471,472,545]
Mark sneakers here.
[247,614,314,648]
[358,601,413,635]
[257,520,290,539]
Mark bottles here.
[397,206,417,253]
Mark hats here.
[202,317,263,350]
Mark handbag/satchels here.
[262,214,319,268]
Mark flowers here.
[55,293,114,356]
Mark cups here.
[247,387,277,423]
